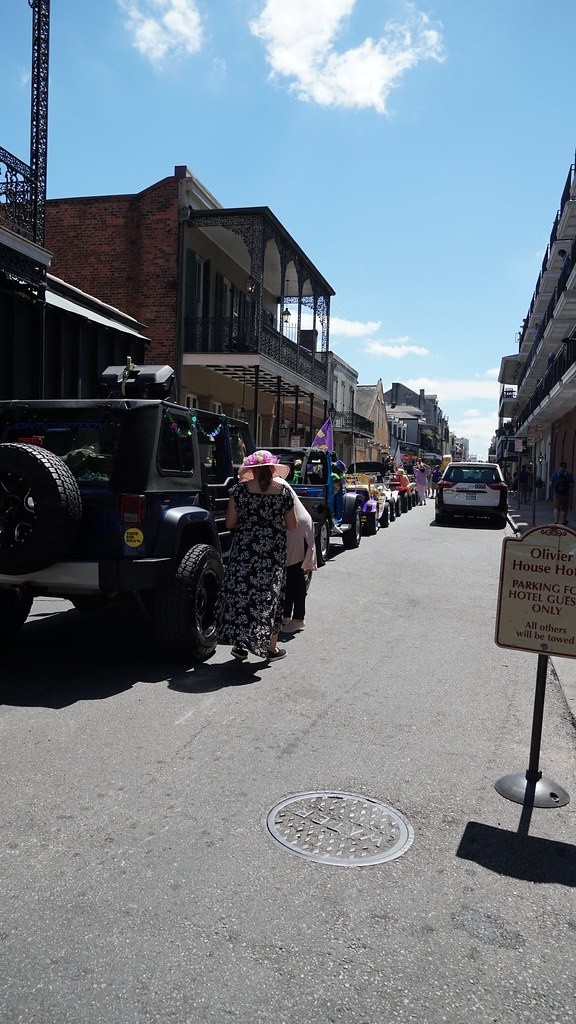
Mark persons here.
[412,460,432,505]
[511,464,534,503]
[429,464,442,499]
[506,472,512,480]
[281,481,317,633]
[550,462,574,525]
[216,450,297,657]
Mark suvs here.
[0,399,257,665]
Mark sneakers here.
[267,647,288,662]
[230,646,249,659]
[281,621,306,632]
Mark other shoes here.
[424,503,427,505]
[418,504,422,506]
[429,496,434,499]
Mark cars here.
[434,462,508,525]
[255,445,419,568]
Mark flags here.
[311,416,334,452]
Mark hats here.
[435,465,440,470]
[238,450,290,480]
[332,459,347,474]
[418,464,426,469]
